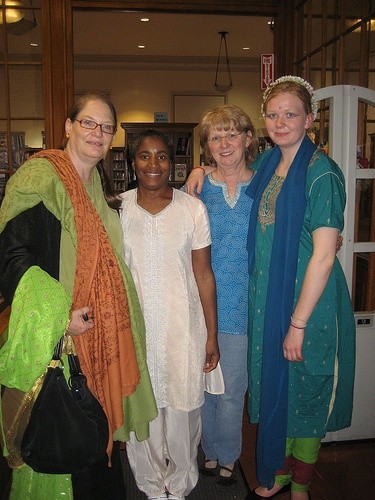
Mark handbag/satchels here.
[21,338,109,474]
[2,367,48,469]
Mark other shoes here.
[289,490,312,500]
[203,455,218,476]
[219,466,234,482]
[245,482,291,500]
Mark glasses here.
[75,119,117,135]
[207,131,245,145]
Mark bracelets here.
[289,311,305,331]
[191,165,206,174]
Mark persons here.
[185,74,357,500]
[108,131,226,500]
[177,105,344,489]
[0,90,158,500]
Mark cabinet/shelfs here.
[0,122,206,205]
